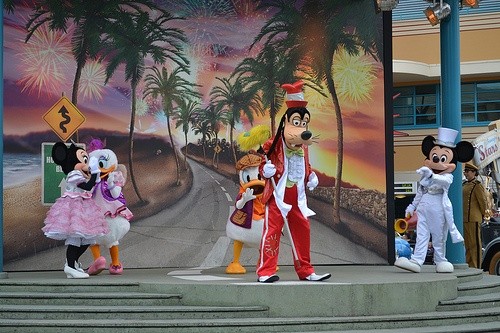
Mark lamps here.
[462,0,480,9]
[423,0,451,27]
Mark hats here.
[463,163,480,171]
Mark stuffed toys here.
[41,139,134,279]
[225,80,332,282]
[394,127,475,273]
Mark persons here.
[462,162,488,270]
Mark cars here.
[407,218,500,275]
